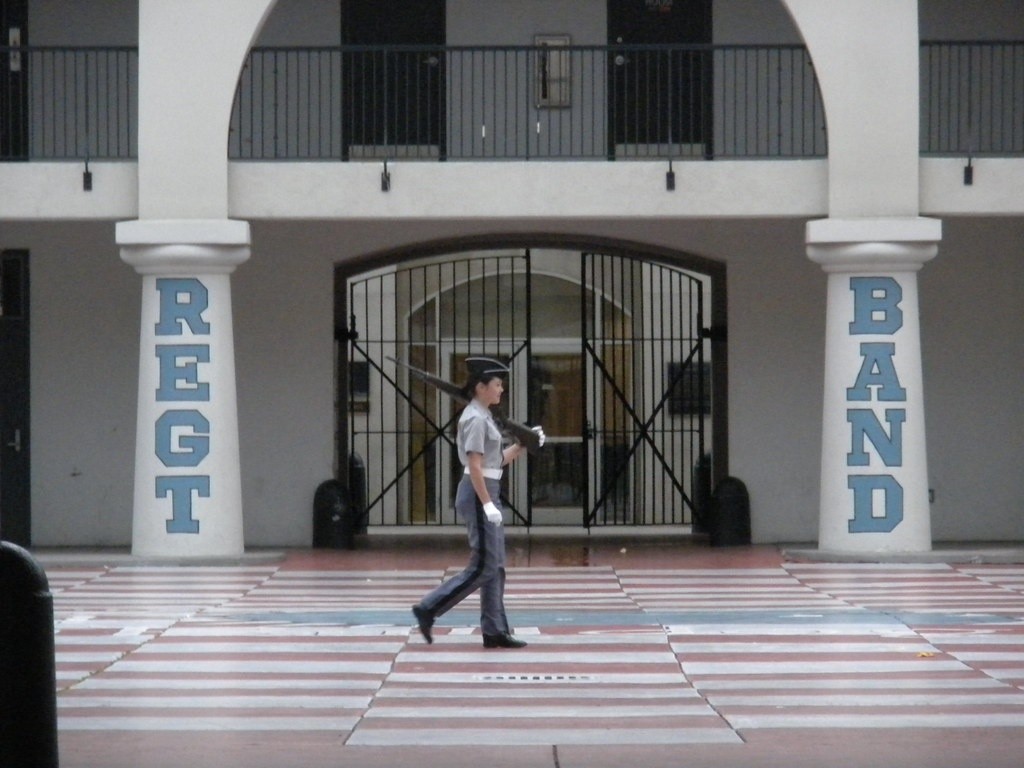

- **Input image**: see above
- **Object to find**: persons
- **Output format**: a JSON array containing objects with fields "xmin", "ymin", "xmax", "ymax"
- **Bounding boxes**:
[{"xmin": 409, "ymin": 357, "xmax": 531, "ymax": 650}]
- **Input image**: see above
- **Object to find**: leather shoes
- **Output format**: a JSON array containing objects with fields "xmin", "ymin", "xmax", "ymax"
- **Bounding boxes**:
[
  {"xmin": 483, "ymin": 631, "xmax": 528, "ymax": 648},
  {"xmin": 412, "ymin": 603, "xmax": 435, "ymax": 644}
]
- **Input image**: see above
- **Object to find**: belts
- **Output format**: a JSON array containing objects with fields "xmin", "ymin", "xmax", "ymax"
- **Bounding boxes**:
[{"xmin": 464, "ymin": 466, "xmax": 503, "ymax": 480}]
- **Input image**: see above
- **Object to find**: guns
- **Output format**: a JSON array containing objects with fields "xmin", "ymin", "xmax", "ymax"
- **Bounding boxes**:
[{"xmin": 384, "ymin": 355, "xmax": 540, "ymax": 451}]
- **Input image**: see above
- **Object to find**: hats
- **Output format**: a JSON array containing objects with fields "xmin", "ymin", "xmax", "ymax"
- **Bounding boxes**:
[{"xmin": 465, "ymin": 354, "xmax": 510, "ymax": 379}]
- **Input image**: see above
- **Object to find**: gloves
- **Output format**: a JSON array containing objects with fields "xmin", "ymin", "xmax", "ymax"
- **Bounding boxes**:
[
  {"xmin": 483, "ymin": 501, "xmax": 502, "ymax": 527},
  {"xmin": 531, "ymin": 426, "xmax": 545, "ymax": 448}
]
